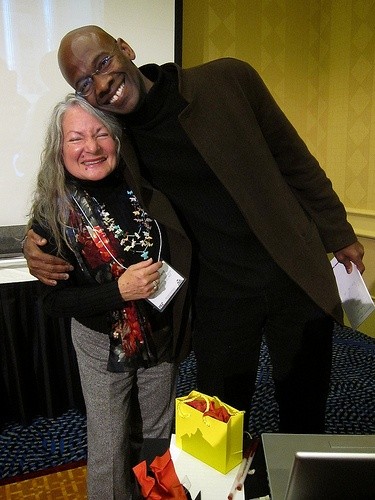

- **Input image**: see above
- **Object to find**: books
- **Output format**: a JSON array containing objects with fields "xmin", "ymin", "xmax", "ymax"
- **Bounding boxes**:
[{"xmin": 329, "ymin": 255, "xmax": 375, "ymax": 330}]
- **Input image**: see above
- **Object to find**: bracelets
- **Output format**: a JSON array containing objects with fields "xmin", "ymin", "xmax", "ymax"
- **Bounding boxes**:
[{"xmin": 19, "ymin": 234, "xmax": 27, "ymax": 254}]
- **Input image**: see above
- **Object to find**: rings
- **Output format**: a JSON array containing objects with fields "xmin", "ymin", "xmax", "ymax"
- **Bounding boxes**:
[{"xmin": 152, "ymin": 280, "xmax": 160, "ymax": 289}]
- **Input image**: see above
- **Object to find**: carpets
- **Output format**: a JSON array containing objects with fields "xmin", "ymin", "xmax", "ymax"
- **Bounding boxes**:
[{"xmin": 0, "ymin": 459, "xmax": 88, "ymax": 500}]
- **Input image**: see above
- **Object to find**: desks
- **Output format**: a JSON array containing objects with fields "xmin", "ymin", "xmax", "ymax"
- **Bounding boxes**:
[{"xmin": 0, "ymin": 256, "xmax": 85, "ymax": 427}]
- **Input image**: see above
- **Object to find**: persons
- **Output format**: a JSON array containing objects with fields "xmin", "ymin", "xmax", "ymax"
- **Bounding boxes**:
[
  {"xmin": 28, "ymin": 93, "xmax": 195, "ymax": 500},
  {"xmin": 18, "ymin": 24, "xmax": 366, "ymax": 480}
]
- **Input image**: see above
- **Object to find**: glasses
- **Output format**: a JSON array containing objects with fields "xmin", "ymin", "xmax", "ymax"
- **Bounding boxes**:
[{"xmin": 75, "ymin": 39, "xmax": 116, "ymax": 97}]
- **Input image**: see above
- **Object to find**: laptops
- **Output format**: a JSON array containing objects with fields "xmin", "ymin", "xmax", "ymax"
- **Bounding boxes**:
[{"xmin": 261, "ymin": 433, "xmax": 375, "ymax": 500}]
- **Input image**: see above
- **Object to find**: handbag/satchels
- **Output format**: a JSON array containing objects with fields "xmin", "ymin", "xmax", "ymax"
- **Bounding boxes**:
[{"xmin": 175, "ymin": 390, "xmax": 246, "ymax": 475}]
